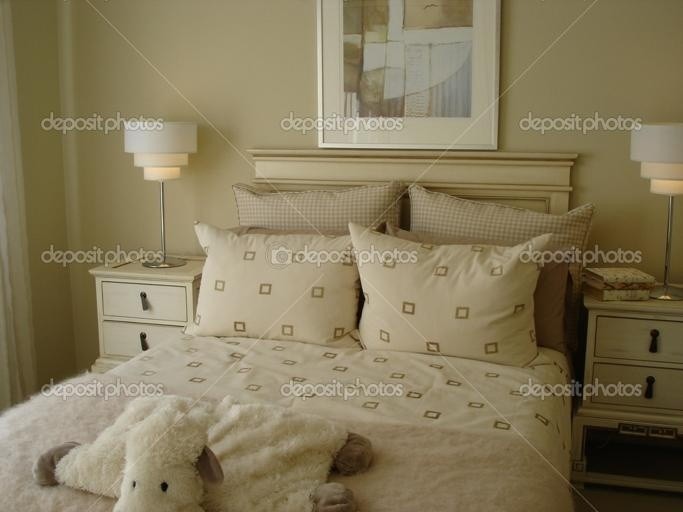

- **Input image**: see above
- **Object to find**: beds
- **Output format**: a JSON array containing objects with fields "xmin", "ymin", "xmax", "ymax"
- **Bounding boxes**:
[{"xmin": 0, "ymin": 149, "xmax": 595, "ymax": 512}]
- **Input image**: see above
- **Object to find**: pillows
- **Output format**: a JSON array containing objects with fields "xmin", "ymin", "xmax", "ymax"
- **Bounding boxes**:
[{"xmin": 182, "ymin": 178, "xmax": 596, "ymax": 370}]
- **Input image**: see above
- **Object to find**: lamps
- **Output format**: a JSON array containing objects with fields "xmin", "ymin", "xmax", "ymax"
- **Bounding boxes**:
[
  {"xmin": 123, "ymin": 117, "xmax": 198, "ymax": 269},
  {"xmin": 626, "ymin": 119, "xmax": 683, "ymax": 302}
]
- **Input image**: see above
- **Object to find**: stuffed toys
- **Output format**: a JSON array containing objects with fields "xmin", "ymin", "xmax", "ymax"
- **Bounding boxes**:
[{"xmin": 34, "ymin": 390, "xmax": 372, "ymax": 512}]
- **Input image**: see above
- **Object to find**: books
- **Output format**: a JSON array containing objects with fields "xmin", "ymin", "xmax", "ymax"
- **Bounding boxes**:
[{"xmin": 582, "ymin": 266, "xmax": 656, "ymax": 301}]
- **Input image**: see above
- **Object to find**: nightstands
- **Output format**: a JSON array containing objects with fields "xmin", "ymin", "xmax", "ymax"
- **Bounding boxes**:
[
  {"xmin": 569, "ymin": 281, "xmax": 682, "ymax": 495},
  {"xmin": 87, "ymin": 253, "xmax": 207, "ymax": 373}
]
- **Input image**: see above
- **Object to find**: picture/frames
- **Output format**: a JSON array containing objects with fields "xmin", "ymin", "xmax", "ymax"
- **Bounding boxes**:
[{"xmin": 314, "ymin": 1, "xmax": 503, "ymax": 152}]
